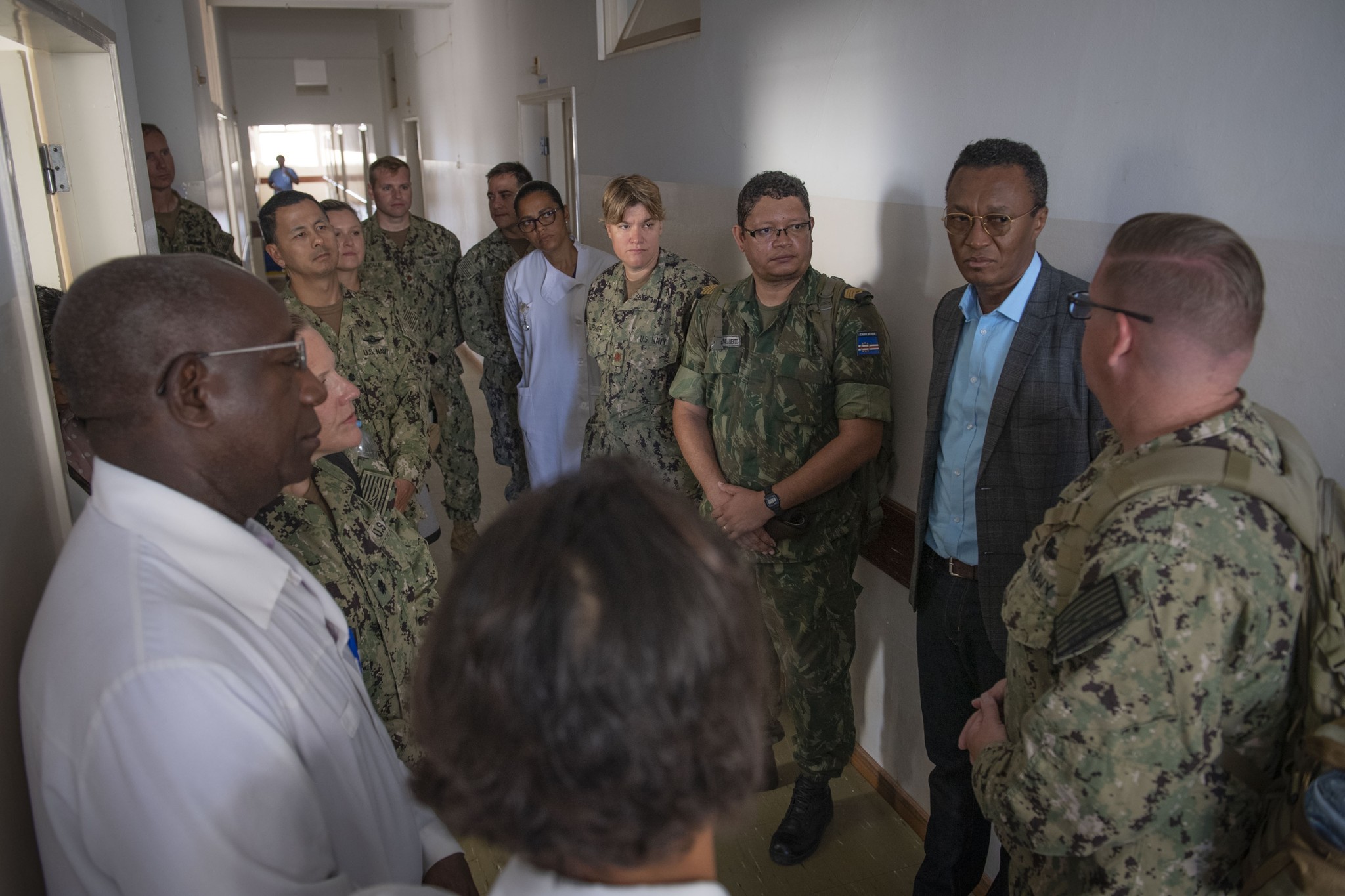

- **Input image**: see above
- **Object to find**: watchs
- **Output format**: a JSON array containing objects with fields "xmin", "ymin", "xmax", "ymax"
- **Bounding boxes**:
[{"xmin": 764, "ymin": 485, "xmax": 783, "ymax": 517}]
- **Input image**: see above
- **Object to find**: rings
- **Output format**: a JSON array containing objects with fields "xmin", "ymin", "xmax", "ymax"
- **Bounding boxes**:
[{"xmin": 724, "ymin": 525, "xmax": 731, "ymax": 533}]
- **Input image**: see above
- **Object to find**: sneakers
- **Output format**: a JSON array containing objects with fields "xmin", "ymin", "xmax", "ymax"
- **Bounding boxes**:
[
  {"xmin": 744, "ymin": 744, "xmax": 778, "ymax": 792},
  {"xmin": 770, "ymin": 776, "xmax": 835, "ymax": 867}
]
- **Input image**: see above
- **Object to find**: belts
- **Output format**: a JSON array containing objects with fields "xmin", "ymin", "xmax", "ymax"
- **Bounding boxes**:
[{"xmin": 921, "ymin": 544, "xmax": 978, "ymax": 581}]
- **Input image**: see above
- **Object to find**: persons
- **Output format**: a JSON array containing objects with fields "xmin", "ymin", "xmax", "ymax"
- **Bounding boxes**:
[
  {"xmin": 402, "ymin": 453, "xmax": 798, "ymax": 895},
  {"xmin": 667, "ymin": 170, "xmax": 893, "ymax": 865},
  {"xmin": 141, "ymin": 122, "xmax": 243, "ymax": 267},
  {"xmin": 905, "ymin": 137, "xmax": 1112, "ymax": 894},
  {"xmin": 256, "ymin": 189, "xmax": 432, "ymax": 536},
  {"xmin": 266, "ymin": 155, "xmax": 299, "ymax": 196},
  {"xmin": 231, "ymin": 308, "xmax": 442, "ymax": 760},
  {"xmin": 502, "ymin": 181, "xmax": 621, "ymax": 495},
  {"xmin": 451, "ymin": 162, "xmax": 537, "ymax": 499},
  {"xmin": 17, "ymin": 251, "xmax": 476, "ymax": 895},
  {"xmin": 320, "ymin": 199, "xmax": 367, "ymax": 293},
  {"xmin": 358, "ymin": 155, "xmax": 487, "ymax": 555},
  {"xmin": 957, "ymin": 213, "xmax": 1320, "ymax": 894},
  {"xmin": 577, "ymin": 176, "xmax": 727, "ymax": 505}
]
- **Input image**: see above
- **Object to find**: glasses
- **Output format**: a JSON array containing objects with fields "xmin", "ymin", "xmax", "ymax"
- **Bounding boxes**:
[
  {"xmin": 517, "ymin": 207, "xmax": 561, "ymax": 234},
  {"xmin": 941, "ymin": 205, "xmax": 1037, "ymax": 237},
  {"xmin": 1066, "ymin": 290, "xmax": 1153, "ymax": 324},
  {"xmin": 740, "ymin": 220, "xmax": 812, "ymax": 243},
  {"xmin": 156, "ymin": 337, "xmax": 306, "ymax": 396}
]
